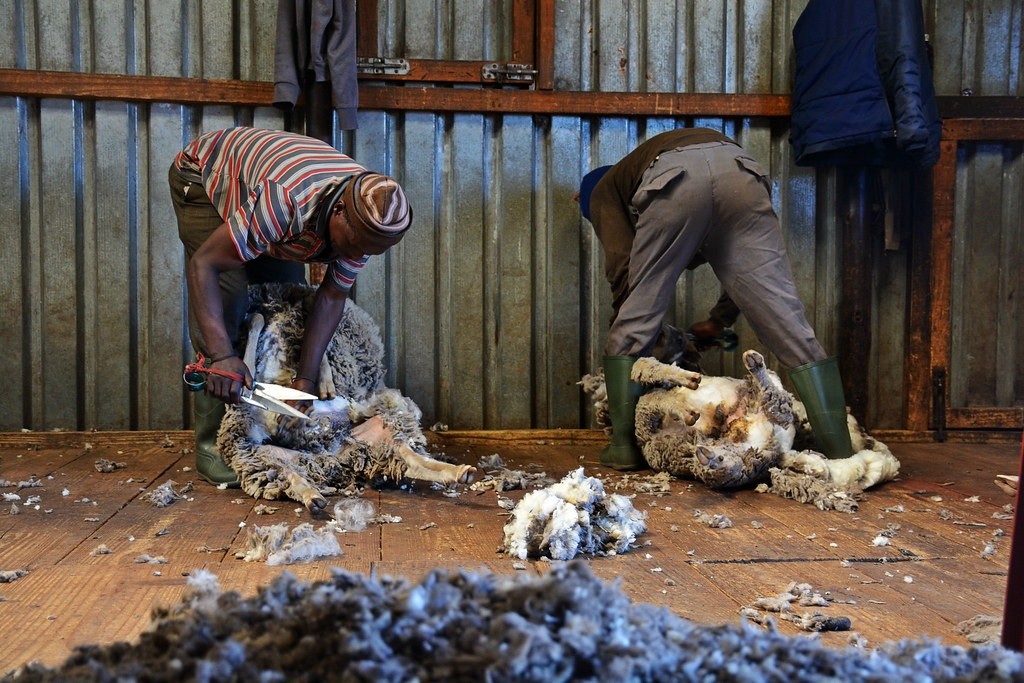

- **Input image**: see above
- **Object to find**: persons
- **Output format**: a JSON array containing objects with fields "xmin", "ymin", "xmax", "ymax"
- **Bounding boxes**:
[
  {"xmin": 579, "ymin": 126, "xmax": 856, "ymax": 470},
  {"xmin": 167, "ymin": 126, "xmax": 413, "ymax": 489}
]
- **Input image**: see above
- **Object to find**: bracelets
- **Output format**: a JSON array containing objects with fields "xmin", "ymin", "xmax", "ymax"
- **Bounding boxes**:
[
  {"xmin": 292, "ymin": 377, "xmax": 317, "ymax": 385},
  {"xmin": 211, "ymin": 351, "xmax": 239, "ymax": 365}
]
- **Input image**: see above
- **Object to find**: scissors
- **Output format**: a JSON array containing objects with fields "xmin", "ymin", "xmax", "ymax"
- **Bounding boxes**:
[
  {"xmin": 663, "ymin": 321, "xmax": 739, "ymax": 352},
  {"xmin": 180, "ymin": 360, "xmax": 320, "ymax": 420}
]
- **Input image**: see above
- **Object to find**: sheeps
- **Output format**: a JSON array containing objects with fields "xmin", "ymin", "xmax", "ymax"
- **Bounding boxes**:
[
  {"xmin": 216, "ymin": 281, "xmax": 477, "ymax": 515},
  {"xmin": 581, "ymin": 348, "xmax": 900, "ymax": 512}
]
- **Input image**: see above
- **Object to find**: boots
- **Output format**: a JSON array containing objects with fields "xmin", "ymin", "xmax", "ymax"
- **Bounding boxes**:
[
  {"xmin": 788, "ymin": 357, "xmax": 854, "ymax": 459},
  {"xmin": 195, "ymin": 357, "xmax": 240, "ymax": 490},
  {"xmin": 599, "ymin": 356, "xmax": 644, "ymax": 470}
]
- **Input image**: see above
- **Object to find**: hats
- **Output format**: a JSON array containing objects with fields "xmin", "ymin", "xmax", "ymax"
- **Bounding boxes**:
[
  {"xmin": 580, "ymin": 165, "xmax": 612, "ymax": 222},
  {"xmin": 344, "ymin": 171, "xmax": 413, "ymax": 255}
]
